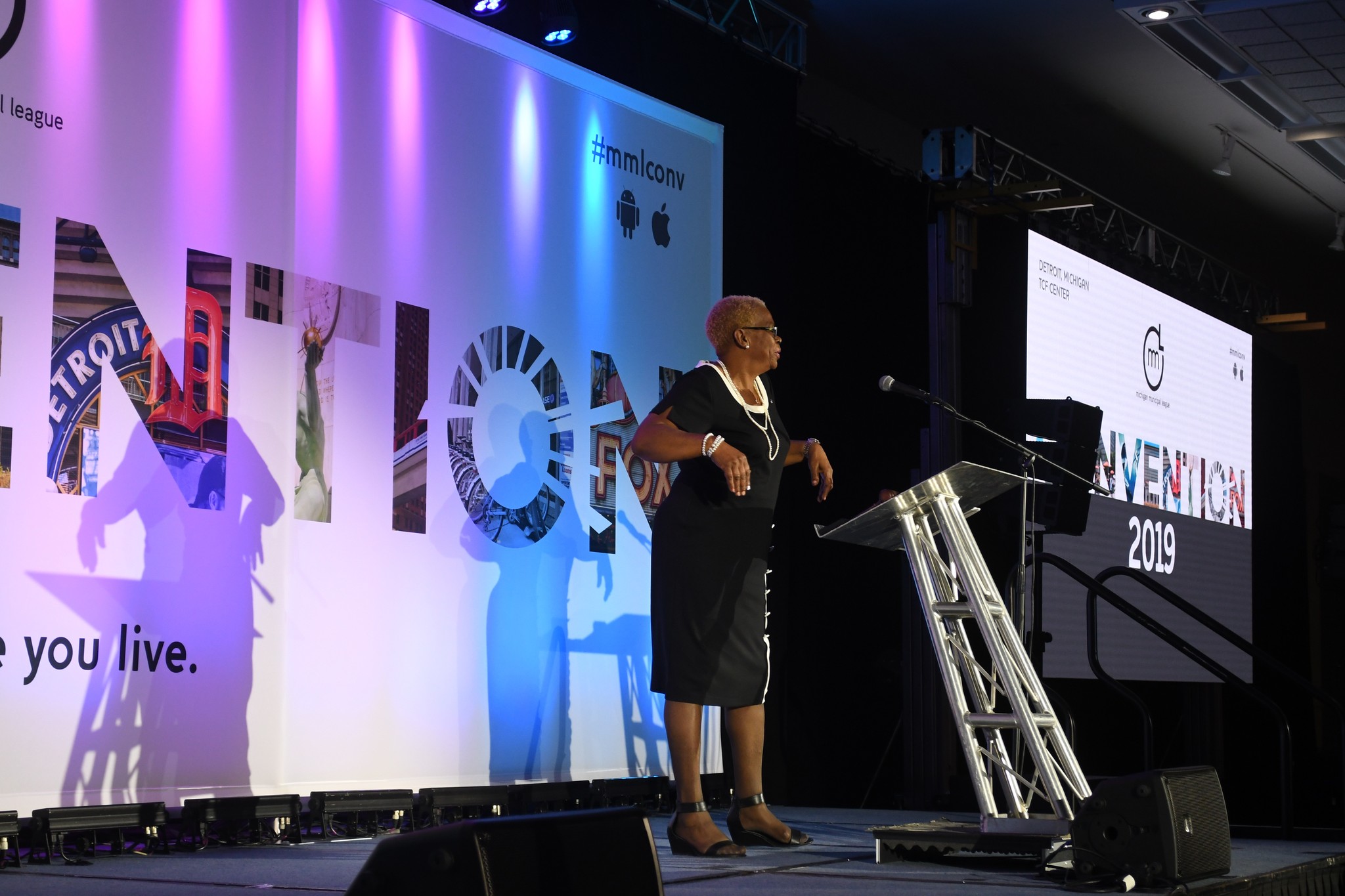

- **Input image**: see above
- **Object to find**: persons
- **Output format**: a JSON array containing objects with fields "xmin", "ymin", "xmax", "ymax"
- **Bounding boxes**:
[
  {"xmin": 633, "ymin": 297, "xmax": 834, "ymax": 858},
  {"xmin": 294, "ymin": 340, "xmax": 328, "ymax": 523}
]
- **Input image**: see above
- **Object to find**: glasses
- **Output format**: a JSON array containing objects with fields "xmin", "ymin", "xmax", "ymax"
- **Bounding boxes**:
[{"xmin": 741, "ymin": 326, "xmax": 778, "ymax": 338}]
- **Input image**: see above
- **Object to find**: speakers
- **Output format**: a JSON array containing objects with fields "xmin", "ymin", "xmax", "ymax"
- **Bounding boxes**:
[
  {"xmin": 1068, "ymin": 764, "xmax": 1233, "ymax": 885},
  {"xmin": 341, "ymin": 806, "xmax": 664, "ymax": 896},
  {"xmin": 1013, "ymin": 398, "xmax": 1104, "ymax": 535}
]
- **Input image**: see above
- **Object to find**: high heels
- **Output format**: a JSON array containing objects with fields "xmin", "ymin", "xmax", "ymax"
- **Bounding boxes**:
[
  {"xmin": 725, "ymin": 794, "xmax": 814, "ymax": 847},
  {"xmin": 666, "ymin": 802, "xmax": 747, "ymax": 860}
]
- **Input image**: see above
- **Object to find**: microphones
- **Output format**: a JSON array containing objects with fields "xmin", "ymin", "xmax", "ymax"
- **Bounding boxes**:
[{"xmin": 879, "ymin": 375, "xmax": 941, "ymax": 403}]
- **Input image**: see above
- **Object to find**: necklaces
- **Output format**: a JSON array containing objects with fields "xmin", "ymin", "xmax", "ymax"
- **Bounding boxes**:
[{"xmin": 716, "ymin": 358, "xmax": 780, "ymax": 460}]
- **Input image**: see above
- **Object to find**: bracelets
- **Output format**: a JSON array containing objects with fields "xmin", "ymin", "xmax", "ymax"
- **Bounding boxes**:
[
  {"xmin": 702, "ymin": 432, "xmax": 725, "ymax": 457},
  {"xmin": 803, "ymin": 438, "xmax": 821, "ymax": 459}
]
